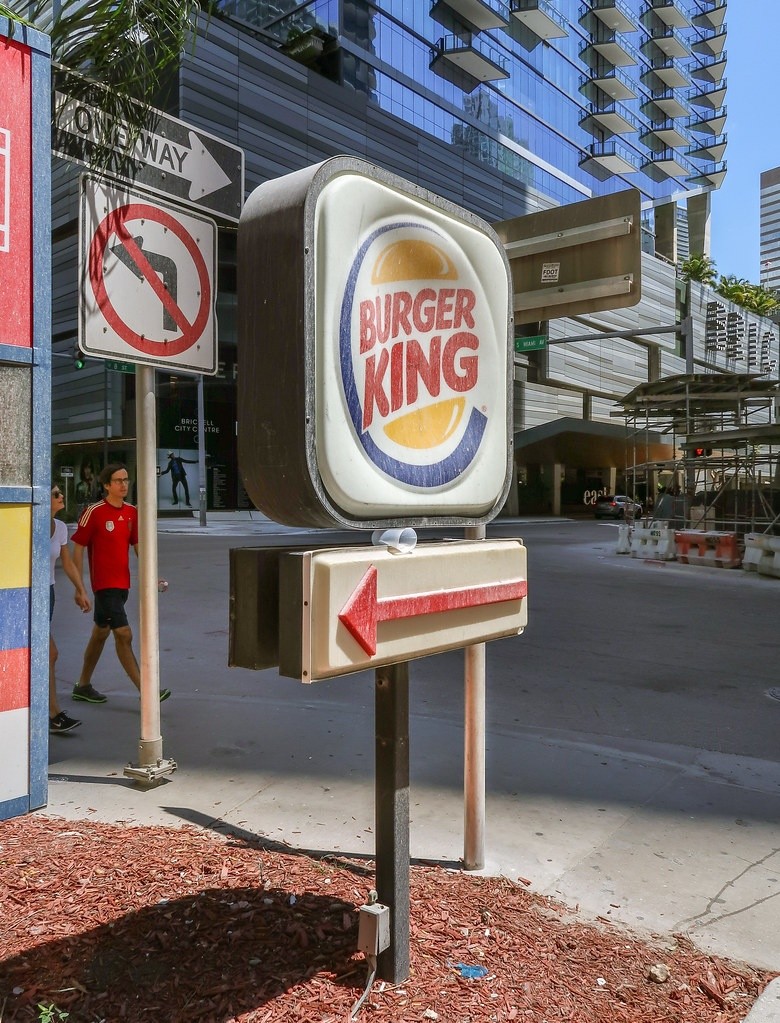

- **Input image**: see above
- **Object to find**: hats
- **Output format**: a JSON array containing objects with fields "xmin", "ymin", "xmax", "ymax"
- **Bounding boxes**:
[{"xmin": 167, "ymin": 450, "xmax": 174, "ymax": 458}]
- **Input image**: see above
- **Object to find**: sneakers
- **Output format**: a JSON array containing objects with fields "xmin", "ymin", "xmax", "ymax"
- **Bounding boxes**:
[
  {"xmin": 48, "ymin": 709, "xmax": 82, "ymax": 733},
  {"xmin": 160, "ymin": 688, "xmax": 172, "ymax": 702},
  {"xmin": 71, "ymin": 681, "xmax": 109, "ymax": 702}
]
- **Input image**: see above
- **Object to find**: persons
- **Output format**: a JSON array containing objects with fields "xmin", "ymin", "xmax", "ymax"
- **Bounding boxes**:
[
  {"xmin": 49, "ymin": 481, "xmax": 91, "ymax": 732},
  {"xmin": 75, "ymin": 458, "xmax": 96, "ymax": 503},
  {"xmin": 161, "ymin": 450, "xmax": 199, "ymax": 508},
  {"xmin": 70, "ymin": 465, "xmax": 171, "ymax": 704}
]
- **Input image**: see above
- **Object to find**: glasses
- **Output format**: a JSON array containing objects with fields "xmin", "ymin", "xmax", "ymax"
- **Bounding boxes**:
[
  {"xmin": 110, "ymin": 477, "xmax": 131, "ymax": 485},
  {"xmin": 51, "ymin": 489, "xmax": 62, "ymax": 499}
]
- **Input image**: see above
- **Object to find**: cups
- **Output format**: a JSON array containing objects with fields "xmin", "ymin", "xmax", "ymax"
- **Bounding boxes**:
[{"xmin": 371, "ymin": 528, "xmax": 417, "ymax": 554}]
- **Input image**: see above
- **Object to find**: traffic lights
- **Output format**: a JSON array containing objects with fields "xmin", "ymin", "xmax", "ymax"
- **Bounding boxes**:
[{"xmin": 694, "ymin": 448, "xmax": 704, "ymax": 456}]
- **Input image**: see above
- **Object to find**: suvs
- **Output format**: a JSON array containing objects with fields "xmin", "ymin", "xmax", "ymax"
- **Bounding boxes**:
[{"xmin": 592, "ymin": 495, "xmax": 643, "ymax": 520}]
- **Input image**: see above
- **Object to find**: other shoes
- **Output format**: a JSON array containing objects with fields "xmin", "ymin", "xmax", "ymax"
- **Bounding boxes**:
[
  {"xmin": 187, "ymin": 503, "xmax": 192, "ymax": 508},
  {"xmin": 172, "ymin": 501, "xmax": 179, "ymax": 505}
]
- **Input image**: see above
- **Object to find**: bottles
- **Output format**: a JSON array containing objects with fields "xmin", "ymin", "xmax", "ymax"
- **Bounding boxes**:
[{"xmin": 158, "ymin": 581, "xmax": 168, "ymax": 592}]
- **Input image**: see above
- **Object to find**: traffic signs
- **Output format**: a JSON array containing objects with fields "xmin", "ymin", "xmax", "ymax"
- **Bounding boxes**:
[{"xmin": 47, "ymin": 60, "xmax": 246, "ymax": 221}]
